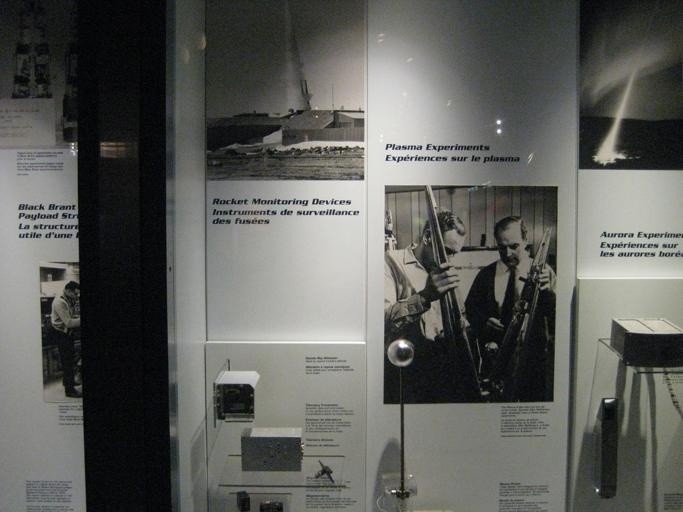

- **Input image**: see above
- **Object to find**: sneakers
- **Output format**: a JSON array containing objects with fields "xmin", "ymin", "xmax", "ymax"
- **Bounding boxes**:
[{"xmin": 63, "ymin": 380, "xmax": 83, "ymax": 397}]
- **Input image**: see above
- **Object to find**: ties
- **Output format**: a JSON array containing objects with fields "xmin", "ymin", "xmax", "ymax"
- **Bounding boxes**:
[{"xmin": 501, "ymin": 265, "xmax": 516, "ymax": 333}]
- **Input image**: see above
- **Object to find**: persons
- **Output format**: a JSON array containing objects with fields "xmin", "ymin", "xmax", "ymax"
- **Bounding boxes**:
[
  {"xmin": 49, "ymin": 280, "xmax": 84, "ymax": 399},
  {"xmin": 462, "ymin": 215, "xmax": 556, "ymax": 402},
  {"xmin": 384, "ymin": 212, "xmax": 480, "ymax": 402}
]
